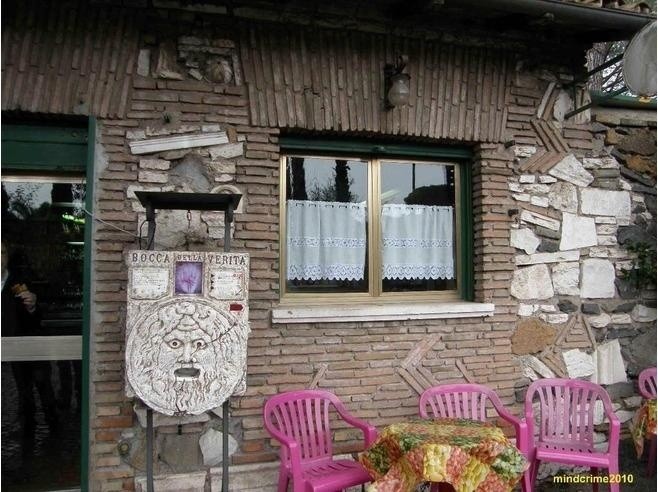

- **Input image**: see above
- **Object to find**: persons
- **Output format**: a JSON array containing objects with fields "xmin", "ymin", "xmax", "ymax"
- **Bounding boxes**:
[
  {"xmin": 1, "ymin": 240, "xmax": 56, "ymax": 337},
  {"xmin": 11, "ymin": 361, "xmax": 57, "ymax": 465},
  {"xmin": 58, "ymin": 361, "xmax": 83, "ymax": 417}
]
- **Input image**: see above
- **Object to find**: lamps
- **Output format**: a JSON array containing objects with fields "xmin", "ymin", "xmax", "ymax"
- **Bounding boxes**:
[{"xmin": 385, "ymin": 71, "xmax": 410, "ymax": 104}]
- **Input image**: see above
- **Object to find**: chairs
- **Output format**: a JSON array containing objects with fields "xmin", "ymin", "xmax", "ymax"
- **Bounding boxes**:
[
  {"xmin": 639, "ymin": 367, "xmax": 657, "ymax": 476},
  {"xmin": 264, "ymin": 389, "xmax": 379, "ymax": 492},
  {"xmin": 526, "ymin": 378, "xmax": 619, "ymax": 492},
  {"xmin": 423, "ymin": 384, "xmax": 532, "ymax": 491}
]
